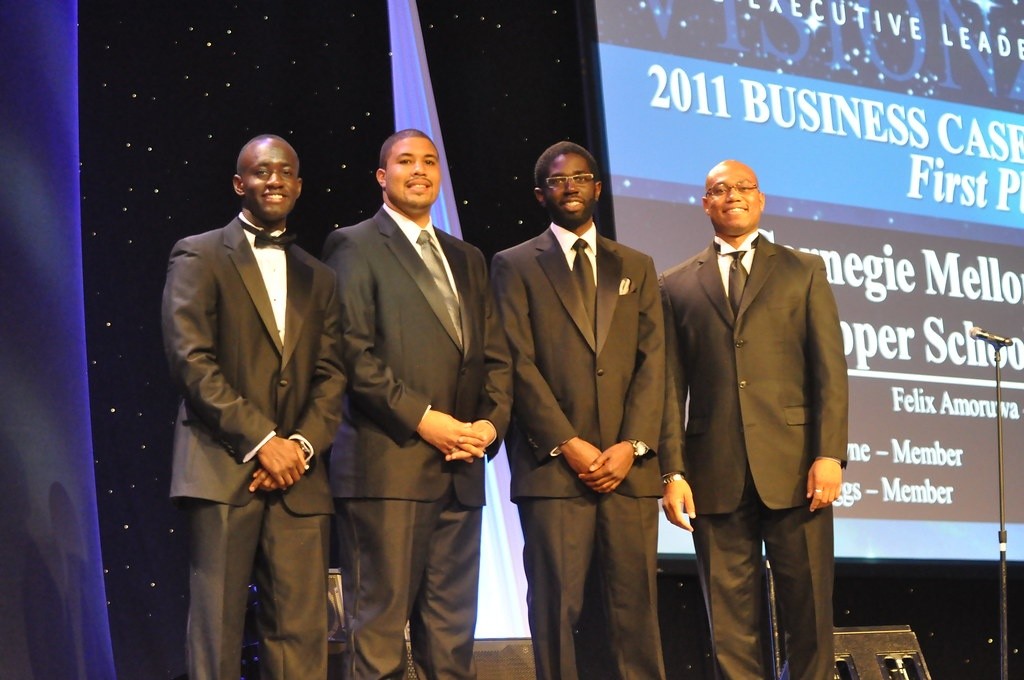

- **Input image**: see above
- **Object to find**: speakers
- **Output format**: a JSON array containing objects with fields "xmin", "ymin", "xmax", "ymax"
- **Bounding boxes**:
[{"xmin": 834, "ymin": 625, "xmax": 933, "ymax": 680}]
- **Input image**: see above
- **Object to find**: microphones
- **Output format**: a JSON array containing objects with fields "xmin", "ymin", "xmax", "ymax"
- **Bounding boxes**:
[{"xmin": 969, "ymin": 327, "xmax": 1013, "ymax": 347}]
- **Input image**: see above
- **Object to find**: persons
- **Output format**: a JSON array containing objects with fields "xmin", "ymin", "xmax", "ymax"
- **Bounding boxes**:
[
  {"xmin": 162, "ymin": 134, "xmax": 347, "ymax": 680},
  {"xmin": 492, "ymin": 140, "xmax": 665, "ymax": 680},
  {"xmin": 658, "ymin": 158, "xmax": 849, "ymax": 680},
  {"xmin": 321, "ymin": 129, "xmax": 515, "ymax": 680}
]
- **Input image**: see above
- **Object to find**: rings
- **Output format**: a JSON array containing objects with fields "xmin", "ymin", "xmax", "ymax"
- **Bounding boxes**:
[
  {"xmin": 815, "ymin": 490, "xmax": 823, "ymax": 492},
  {"xmin": 453, "ymin": 447, "xmax": 457, "ymax": 453}
]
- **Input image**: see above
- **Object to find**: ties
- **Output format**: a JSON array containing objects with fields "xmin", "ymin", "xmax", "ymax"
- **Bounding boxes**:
[
  {"xmin": 416, "ymin": 230, "xmax": 464, "ymax": 350},
  {"xmin": 712, "ymin": 236, "xmax": 759, "ymax": 322},
  {"xmin": 571, "ymin": 238, "xmax": 597, "ymax": 341}
]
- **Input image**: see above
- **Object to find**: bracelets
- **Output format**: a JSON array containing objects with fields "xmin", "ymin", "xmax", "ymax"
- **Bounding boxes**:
[
  {"xmin": 664, "ymin": 473, "xmax": 684, "ymax": 484},
  {"xmin": 300, "ymin": 441, "xmax": 311, "ymax": 453}
]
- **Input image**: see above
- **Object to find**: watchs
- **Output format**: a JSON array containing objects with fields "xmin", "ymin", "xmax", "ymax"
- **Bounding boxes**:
[{"xmin": 631, "ymin": 439, "xmax": 648, "ymax": 457}]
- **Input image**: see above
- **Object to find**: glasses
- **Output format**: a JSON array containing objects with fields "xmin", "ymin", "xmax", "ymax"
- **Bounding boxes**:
[
  {"xmin": 705, "ymin": 180, "xmax": 758, "ymax": 200},
  {"xmin": 540, "ymin": 173, "xmax": 594, "ymax": 189}
]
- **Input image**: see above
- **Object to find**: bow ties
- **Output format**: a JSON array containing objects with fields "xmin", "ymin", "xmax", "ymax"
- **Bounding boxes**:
[{"xmin": 237, "ymin": 217, "xmax": 298, "ymax": 252}]
